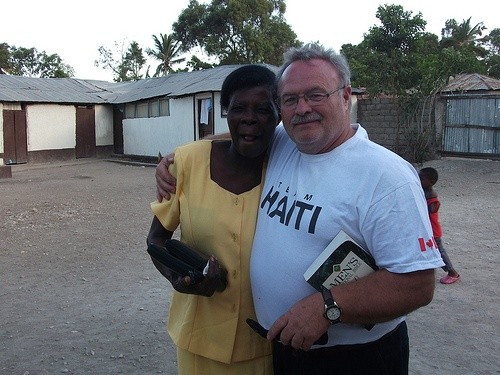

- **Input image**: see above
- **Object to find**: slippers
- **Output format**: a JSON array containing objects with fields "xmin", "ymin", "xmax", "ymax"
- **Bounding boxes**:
[{"xmin": 441, "ymin": 273, "xmax": 462, "ymax": 284}]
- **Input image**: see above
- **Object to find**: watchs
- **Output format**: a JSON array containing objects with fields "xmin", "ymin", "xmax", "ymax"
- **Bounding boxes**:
[{"xmin": 320, "ymin": 289, "xmax": 343, "ymax": 326}]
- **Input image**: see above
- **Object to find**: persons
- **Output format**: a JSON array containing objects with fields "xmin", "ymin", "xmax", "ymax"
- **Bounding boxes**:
[
  {"xmin": 155, "ymin": 42, "xmax": 447, "ymax": 375},
  {"xmin": 416, "ymin": 167, "xmax": 462, "ymax": 284},
  {"xmin": 144, "ymin": 64, "xmax": 283, "ymax": 375}
]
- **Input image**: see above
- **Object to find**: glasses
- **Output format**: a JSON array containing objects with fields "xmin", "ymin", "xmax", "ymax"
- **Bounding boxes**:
[{"xmin": 276, "ymin": 87, "xmax": 345, "ymax": 110}]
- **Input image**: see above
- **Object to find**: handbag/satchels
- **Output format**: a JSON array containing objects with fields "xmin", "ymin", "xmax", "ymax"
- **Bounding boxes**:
[{"xmin": 147, "ymin": 239, "xmax": 228, "ymax": 292}]
